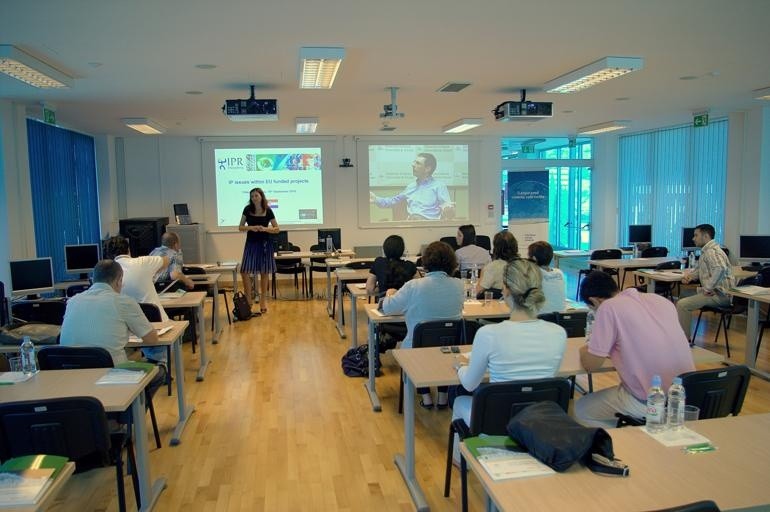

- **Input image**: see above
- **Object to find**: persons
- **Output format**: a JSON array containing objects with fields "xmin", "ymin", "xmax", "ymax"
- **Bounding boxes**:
[
  {"xmin": 102, "ymin": 235, "xmax": 171, "ymax": 323},
  {"xmin": 570, "ymin": 270, "xmax": 695, "ymax": 428},
  {"xmin": 58, "ymin": 258, "xmax": 166, "ymax": 413},
  {"xmin": 456, "ymin": 225, "xmax": 492, "ymax": 269},
  {"xmin": 238, "ymin": 187, "xmax": 279, "ymax": 314},
  {"xmin": 366, "ymin": 235, "xmax": 422, "ymax": 297},
  {"xmin": 453, "ymin": 262, "xmax": 567, "ymax": 470},
  {"xmin": 503, "ymin": 241, "xmax": 565, "ymax": 313},
  {"xmin": 476, "ymin": 230, "xmax": 521, "ymax": 323},
  {"xmin": 675, "ymin": 223, "xmax": 736, "ymax": 346},
  {"xmin": 370, "ymin": 152, "xmax": 456, "ymax": 221},
  {"xmin": 147, "ymin": 231, "xmax": 195, "ymax": 290},
  {"xmin": 383, "ymin": 241, "xmax": 466, "ymax": 410}
]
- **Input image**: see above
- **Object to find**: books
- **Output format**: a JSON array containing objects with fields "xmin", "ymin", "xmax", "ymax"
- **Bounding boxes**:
[
  {"xmin": 96, "ymin": 361, "xmax": 156, "ymax": 385},
  {"xmin": 160, "ymin": 291, "xmax": 186, "ymax": 299},
  {"xmin": 463, "ymin": 436, "xmax": 557, "ymax": 482},
  {"xmin": 0, "ymin": 454, "xmax": 78, "ymax": 506}
]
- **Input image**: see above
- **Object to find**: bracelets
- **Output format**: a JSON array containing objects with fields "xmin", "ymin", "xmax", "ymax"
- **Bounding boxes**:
[
  {"xmin": 456, "ymin": 362, "xmax": 465, "ymax": 372},
  {"xmin": 388, "ymin": 294, "xmax": 392, "ymax": 296}
]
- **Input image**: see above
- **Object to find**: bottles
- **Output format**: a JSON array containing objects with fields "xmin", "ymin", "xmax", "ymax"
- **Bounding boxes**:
[
  {"xmin": 666, "ymin": 378, "xmax": 686, "ymax": 431},
  {"xmin": 177, "ymin": 249, "xmax": 184, "ymax": 265},
  {"xmin": 632, "ymin": 242, "xmax": 638, "ymax": 258},
  {"xmin": 645, "ymin": 374, "xmax": 667, "ymax": 434},
  {"xmin": 461, "ymin": 262, "xmax": 479, "ymax": 303},
  {"xmin": 583, "ymin": 309, "xmax": 596, "ymax": 346},
  {"xmin": 20, "ymin": 336, "xmax": 36, "ymax": 374},
  {"xmin": 688, "ymin": 252, "xmax": 695, "ymax": 270}
]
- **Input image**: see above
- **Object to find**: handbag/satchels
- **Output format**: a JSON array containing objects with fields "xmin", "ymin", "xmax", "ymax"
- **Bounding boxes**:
[
  {"xmin": 233, "ymin": 291, "xmax": 251, "ymax": 321},
  {"xmin": 507, "ymin": 400, "xmax": 614, "ymax": 471},
  {"xmin": 342, "ymin": 344, "xmax": 368, "ymax": 377}
]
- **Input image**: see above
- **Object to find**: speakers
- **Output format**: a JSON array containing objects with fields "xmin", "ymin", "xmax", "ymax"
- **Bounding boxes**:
[{"xmin": 119, "ymin": 217, "xmax": 170, "ymax": 258}]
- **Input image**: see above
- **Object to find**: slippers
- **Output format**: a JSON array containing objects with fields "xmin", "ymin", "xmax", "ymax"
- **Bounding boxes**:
[
  {"xmin": 420, "ymin": 400, "xmax": 433, "ymax": 408},
  {"xmin": 437, "ymin": 400, "xmax": 448, "ymax": 408}
]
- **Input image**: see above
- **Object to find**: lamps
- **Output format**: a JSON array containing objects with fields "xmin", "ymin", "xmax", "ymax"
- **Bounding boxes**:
[
  {"xmin": 121, "ymin": 118, "xmax": 166, "ymax": 135},
  {"xmin": 441, "ymin": 118, "xmax": 483, "ymax": 136},
  {"xmin": 751, "ymin": 88, "xmax": 770, "ymax": 101},
  {"xmin": 520, "ymin": 139, "xmax": 546, "ymax": 146},
  {"xmin": 579, "ymin": 121, "xmax": 631, "ymax": 136},
  {"xmin": 295, "ymin": 117, "xmax": 317, "ymax": 133},
  {"xmin": 544, "ymin": 57, "xmax": 643, "ymax": 96},
  {"xmin": 0, "ymin": 45, "xmax": 75, "ymax": 91},
  {"xmin": 298, "ymin": 47, "xmax": 345, "ymax": 91}
]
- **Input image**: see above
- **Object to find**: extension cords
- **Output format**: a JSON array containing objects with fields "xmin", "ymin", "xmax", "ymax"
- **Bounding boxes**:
[{"xmin": 315, "ymin": 295, "xmax": 332, "ymax": 300}]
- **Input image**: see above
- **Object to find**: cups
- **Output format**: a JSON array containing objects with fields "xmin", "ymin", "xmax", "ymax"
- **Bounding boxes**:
[
  {"xmin": 679, "ymin": 405, "xmax": 701, "ymax": 440},
  {"xmin": 662, "ymin": 407, "xmax": 681, "ymax": 442},
  {"xmin": 484, "ymin": 291, "xmax": 493, "ymax": 304},
  {"xmin": 8, "ymin": 357, "xmax": 24, "ymax": 380}
]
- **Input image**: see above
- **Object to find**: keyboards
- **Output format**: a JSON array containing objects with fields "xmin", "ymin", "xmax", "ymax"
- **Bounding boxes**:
[
  {"xmin": 279, "ymin": 251, "xmax": 293, "ymax": 254},
  {"xmin": 312, "ymin": 250, "xmax": 327, "ymax": 252}
]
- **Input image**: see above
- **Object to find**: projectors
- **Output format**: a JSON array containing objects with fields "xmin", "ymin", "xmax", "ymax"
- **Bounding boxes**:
[
  {"xmin": 222, "ymin": 99, "xmax": 279, "ymax": 122},
  {"xmin": 495, "ymin": 102, "xmax": 554, "ymax": 122}
]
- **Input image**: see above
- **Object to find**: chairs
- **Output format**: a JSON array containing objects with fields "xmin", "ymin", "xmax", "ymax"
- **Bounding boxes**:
[
  {"xmin": 443, "ymin": 375, "xmax": 578, "ymax": 511},
  {"xmin": 12, "ymin": 301, "xmax": 173, "ymax": 396},
  {"xmin": 2, "ymin": 346, "xmax": 163, "ymax": 449},
  {"xmin": 309, "ymin": 245, "xmax": 342, "ymax": 299},
  {"xmin": 0, "ymin": 395, "xmax": 142, "ymax": 512},
  {"xmin": 614, "ymin": 361, "xmax": 753, "ymax": 431},
  {"xmin": 67, "ymin": 267, "xmax": 232, "ymax": 353},
  {"xmin": 377, "ymin": 268, "xmax": 595, "ymax": 414},
  {"xmin": 576, "ymin": 245, "xmax": 769, "ymax": 364},
  {"xmin": 271, "ymin": 246, "xmax": 308, "ymax": 299},
  {"xmin": 330, "ymin": 255, "xmax": 431, "ymax": 320}
]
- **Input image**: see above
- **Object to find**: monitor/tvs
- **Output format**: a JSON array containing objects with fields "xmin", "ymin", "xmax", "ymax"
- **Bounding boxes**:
[
  {"xmin": 318, "ymin": 228, "xmax": 341, "ymax": 252},
  {"xmin": 681, "ymin": 227, "xmax": 702, "ymax": 260},
  {"xmin": 64, "ymin": 244, "xmax": 99, "ymax": 281},
  {"xmin": 629, "ymin": 225, "xmax": 652, "ymax": 245},
  {"xmin": 271, "ymin": 231, "xmax": 289, "ymax": 256},
  {"xmin": 9, "ymin": 257, "xmax": 55, "ymax": 302},
  {"xmin": 737, "ymin": 234, "xmax": 770, "ymax": 270}
]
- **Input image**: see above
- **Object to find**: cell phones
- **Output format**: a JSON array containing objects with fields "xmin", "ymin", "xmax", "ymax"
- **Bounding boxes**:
[
  {"xmin": 441, "ymin": 347, "xmax": 451, "ymax": 353},
  {"xmin": 451, "ymin": 347, "xmax": 460, "ymax": 353}
]
